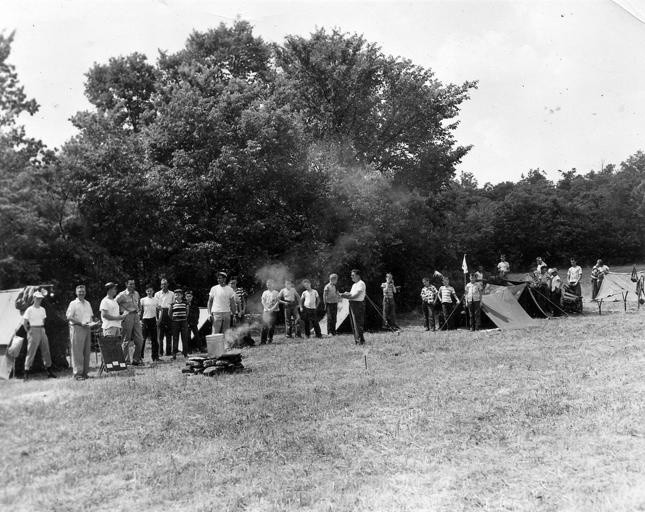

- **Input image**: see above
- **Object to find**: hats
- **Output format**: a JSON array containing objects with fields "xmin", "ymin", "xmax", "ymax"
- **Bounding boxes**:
[
  {"xmin": 105, "ymin": 282, "xmax": 118, "ymax": 289},
  {"xmin": 32, "ymin": 292, "xmax": 44, "ymax": 298}
]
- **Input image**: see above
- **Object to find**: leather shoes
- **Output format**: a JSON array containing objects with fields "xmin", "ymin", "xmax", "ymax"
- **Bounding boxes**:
[
  {"xmin": 24, "ymin": 371, "xmax": 29, "ymax": 380},
  {"xmin": 47, "ymin": 367, "xmax": 57, "ymax": 377}
]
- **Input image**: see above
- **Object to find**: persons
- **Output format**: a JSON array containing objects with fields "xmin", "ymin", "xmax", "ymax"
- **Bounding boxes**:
[
  {"xmin": 22, "ymin": 291, "xmax": 58, "ymax": 382},
  {"xmin": 64, "ymin": 284, "xmax": 97, "ymax": 382},
  {"xmin": 98, "ymin": 255, "xmax": 609, "ymax": 367}
]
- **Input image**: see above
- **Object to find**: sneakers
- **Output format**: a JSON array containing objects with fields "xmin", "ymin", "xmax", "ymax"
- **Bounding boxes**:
[
  {"xmin": 131, "ymin": 354, "xmax": 163, "ymax": 366},
  {"xmin": 165, "ymin": 351, "xmax": 206, "ymax": 360},
  {"xmin": 76, "ymin": 375, "xmax": 94, "ymax": 380}
]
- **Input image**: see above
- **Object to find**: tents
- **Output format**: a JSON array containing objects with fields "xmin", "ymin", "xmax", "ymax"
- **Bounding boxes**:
[
  {"xmin": 591, "ymin": 269, "xmax": 645, "ymax": 302},
  {"xmin": 0, "ymin": 285, "xmax": 69, "ymax": 380}
]
